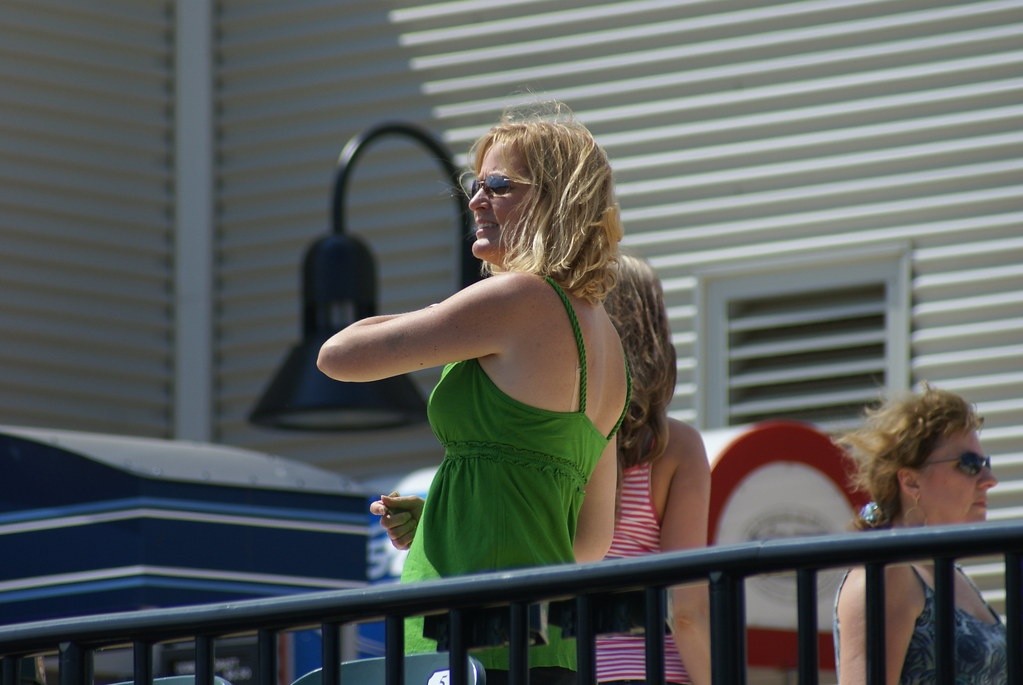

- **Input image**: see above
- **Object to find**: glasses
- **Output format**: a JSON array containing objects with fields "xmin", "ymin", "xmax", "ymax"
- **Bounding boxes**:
[
  {"xmin": 472, "ymin": 174, "xmax": 538, "ymax": 199},
  {"xmin": 923, "ymin": 452, "xmax": 991, "ymax": 476}
]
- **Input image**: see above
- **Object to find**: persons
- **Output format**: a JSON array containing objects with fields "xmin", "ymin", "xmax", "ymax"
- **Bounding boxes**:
[
  {"xmin": 315, "ymin": 120, "xmax": 632, "ymax": 685},
  {"xmin": 593, "ymin": 255, "xmax": 712, "ymax": 685},
  {"xmin": 832, "ymin": 389, "xmax": 1005, "ymax": 685}
]
